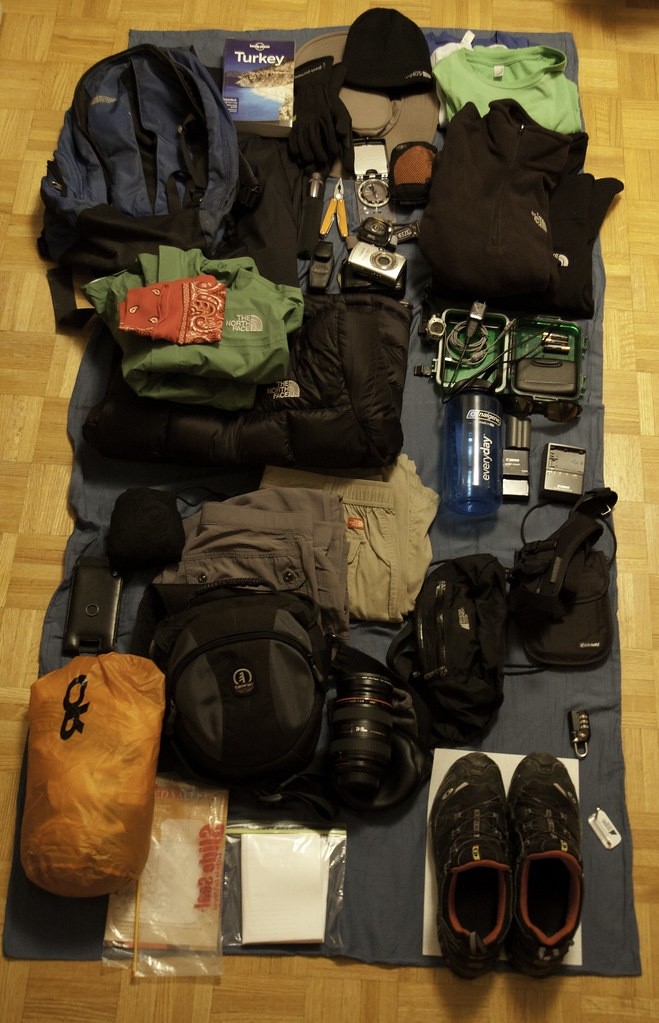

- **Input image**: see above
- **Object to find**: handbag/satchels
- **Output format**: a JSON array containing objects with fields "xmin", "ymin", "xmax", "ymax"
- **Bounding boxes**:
[
  {"xmin": 108, "ymin": 486, "xmax": 186, "ymax": 569},
  {"xmin": 20, "ymin": 650, "xmax": 163, "ymax": 898},
  {"xmin": 506, "ymin": 486, "xmax": 615, "ymax": 667},
  {"xmin": 388, "ymin": 551, "xmax": 511, "ymax": 741}
]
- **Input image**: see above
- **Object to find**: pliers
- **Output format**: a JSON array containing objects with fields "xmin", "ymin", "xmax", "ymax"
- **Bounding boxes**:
[{"xmin": 319, "ymin": 178, "xmax": 349, "ymax": 239}]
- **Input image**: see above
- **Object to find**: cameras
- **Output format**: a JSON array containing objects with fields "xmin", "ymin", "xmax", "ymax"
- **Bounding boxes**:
[{"xmin": 347, "ymin": 241, "xmax": 407, "ymax": 288}]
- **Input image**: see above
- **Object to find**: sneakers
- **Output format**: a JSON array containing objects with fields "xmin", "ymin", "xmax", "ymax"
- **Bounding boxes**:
[
  {"xmin": 430, "ymin": 753, "xmax": 515, "ymax": 978},
  {"xmin": 508, "ymin": 750, "xmax": 581, "ymax": 976}
]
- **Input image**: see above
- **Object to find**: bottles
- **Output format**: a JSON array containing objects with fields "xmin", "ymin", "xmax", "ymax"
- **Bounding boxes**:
[{"xmin": 441, "ymin": 378, "xmax": 503, "ymax": 516}]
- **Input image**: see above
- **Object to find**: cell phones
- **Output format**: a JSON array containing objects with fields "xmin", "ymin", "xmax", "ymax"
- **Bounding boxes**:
[{"xmin": 61, "ymin": 564, "xmax": 122, "ymax": 655}]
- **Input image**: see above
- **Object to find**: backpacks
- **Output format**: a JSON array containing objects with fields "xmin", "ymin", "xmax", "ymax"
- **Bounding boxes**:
[
  {"xmin": 35, "ymin": 46, "xmax": 240, "ymax": 272},
  {"xmin": 145, "ymin": 575, "xmax": 323, "ymax": 803}
]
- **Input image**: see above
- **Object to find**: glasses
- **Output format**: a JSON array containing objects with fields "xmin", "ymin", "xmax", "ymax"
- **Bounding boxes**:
[{"xmin": 502, "ymin": 392, "xmax": 585, "ymax": 422}]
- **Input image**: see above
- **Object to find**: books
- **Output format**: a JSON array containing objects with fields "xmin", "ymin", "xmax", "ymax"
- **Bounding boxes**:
[{"xmin": 220, "ymin": 38, "xmax": 295, "ymax": 138}]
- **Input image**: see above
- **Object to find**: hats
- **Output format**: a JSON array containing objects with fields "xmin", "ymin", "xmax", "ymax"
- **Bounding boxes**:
[{"xmin": 330, "ymin": 8, "xmax": 434, "ymax": 96}]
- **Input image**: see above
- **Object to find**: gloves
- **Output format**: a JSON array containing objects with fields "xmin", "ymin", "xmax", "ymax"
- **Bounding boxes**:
[{"xmin": 288, "ymin": 55, "xmax": 354, "ymax": 174}]
